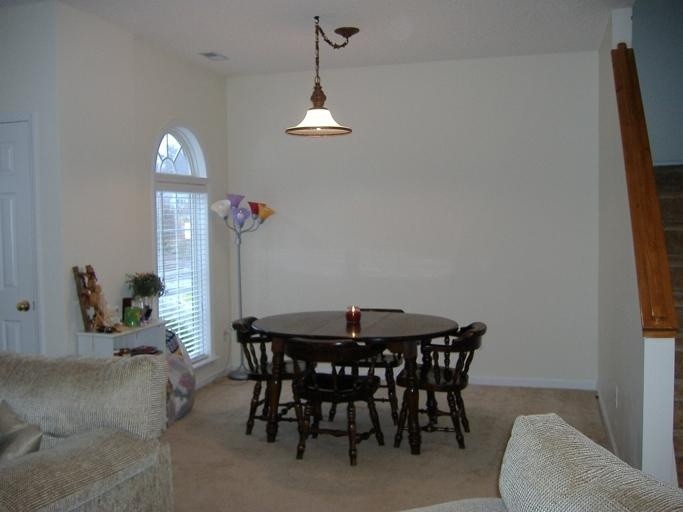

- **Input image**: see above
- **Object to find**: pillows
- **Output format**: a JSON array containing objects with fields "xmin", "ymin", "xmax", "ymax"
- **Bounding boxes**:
[{"xmin": 1, "ymin": 399, "xmax": 44, "ymax": 463}]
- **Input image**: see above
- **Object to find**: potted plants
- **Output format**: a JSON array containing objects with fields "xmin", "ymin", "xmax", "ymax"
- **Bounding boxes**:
[{"xmin": 124, "ymin": 270, "xmax": 165, "ymax": 326}]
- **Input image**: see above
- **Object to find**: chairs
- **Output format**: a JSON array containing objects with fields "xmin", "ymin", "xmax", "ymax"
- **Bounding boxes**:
[
  {"xmin": 405, "ymin": 413, "xmax": 683, "ymax": 511},
  {"xmin": 0, "ymin": 347, "xmax": 174, "ymax": 512}
]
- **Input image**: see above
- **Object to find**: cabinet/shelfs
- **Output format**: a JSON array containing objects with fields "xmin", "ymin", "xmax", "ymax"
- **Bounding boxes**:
[{"xmin": 75, "ymin": 319, "xmax": 171, "ymax": 356}]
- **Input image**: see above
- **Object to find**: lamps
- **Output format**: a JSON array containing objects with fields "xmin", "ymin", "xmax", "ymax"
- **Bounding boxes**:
[
  {"xmin": 210, "ymin": 193, "xmax": 276, "ymax": 380},
  {"xmin": 285, "ymin": 15, "xmax": 360, "ymax": 137}
]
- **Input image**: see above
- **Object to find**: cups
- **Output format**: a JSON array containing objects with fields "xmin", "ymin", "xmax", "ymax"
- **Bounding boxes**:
[
  {"xmin": 125, "ymin": 306, "xmax": 141, "ymax": 328},
  {"xmin": 344, "ymin": 305, "xmax": 361, "ymax": 326}
]
